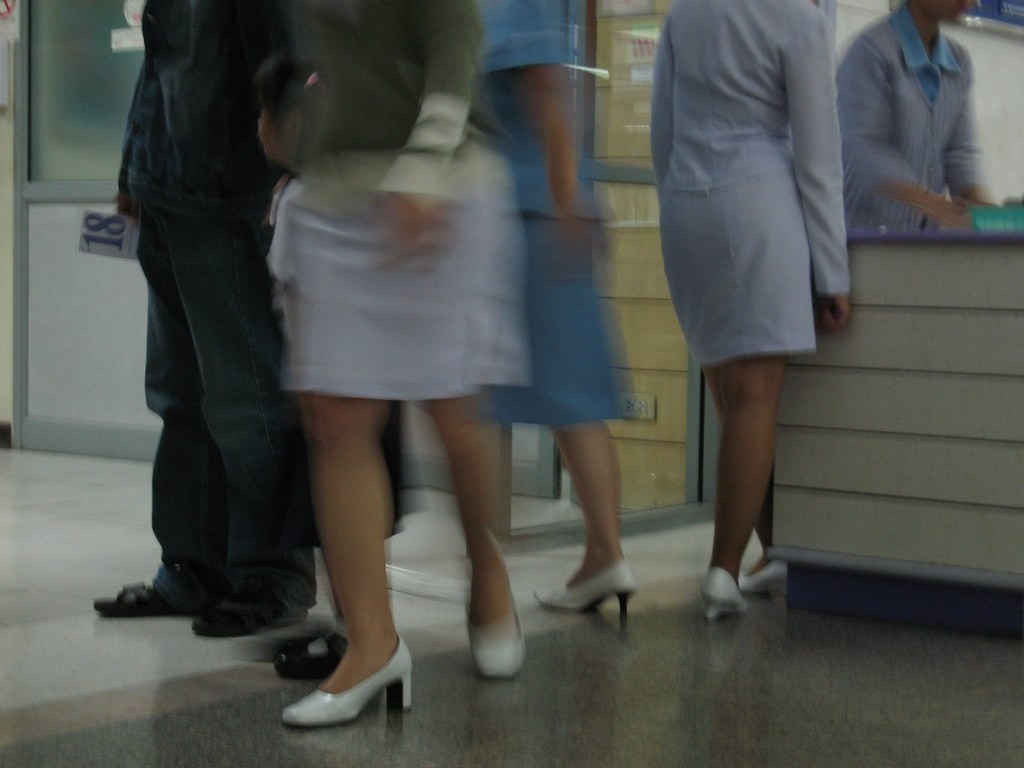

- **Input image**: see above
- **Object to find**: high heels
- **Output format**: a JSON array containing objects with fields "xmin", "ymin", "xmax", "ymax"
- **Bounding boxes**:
[
  {"xmin": 739, "ymin": 559, "xmax": 787, "ymax": 596},
  {"xmin": 281, "ymin": 632, "xmax": 411, "ymax": 726},
  {"xmin": 700, "ymin": 566, "xmax": 747, "ymax": 617},
  {"xmin": 466, "ymin": 597, "xmax": 525, "ymax": 680},
  {"xmin": 533, "ymin": 561, "xmax": 635, "ymax": 615}
]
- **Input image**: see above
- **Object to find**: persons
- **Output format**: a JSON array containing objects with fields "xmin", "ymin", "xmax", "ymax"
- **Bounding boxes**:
[
  {"xmin": 94, "ymin": 0, "xmax": 634, "ymax": 726},
  {"xmin": 650, "ymin": 0, "xmax": 852, "ymax": 620},
  {"xmin": 838, "ymin": 0, "xmax": 998, "ymax": 235}
]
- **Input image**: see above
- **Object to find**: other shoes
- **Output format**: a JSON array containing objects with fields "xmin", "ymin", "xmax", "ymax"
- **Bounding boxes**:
[
  {"xmin": 94, "ymin": 583, "xmax": 189, "ymax": 616},
  {"xmin": 192, "ymin": 596, "xmax": 310, "ymax": 635},
  {"xmin": 272, "ymin": 630, "xmax": 350, "ymax": 677}
]
